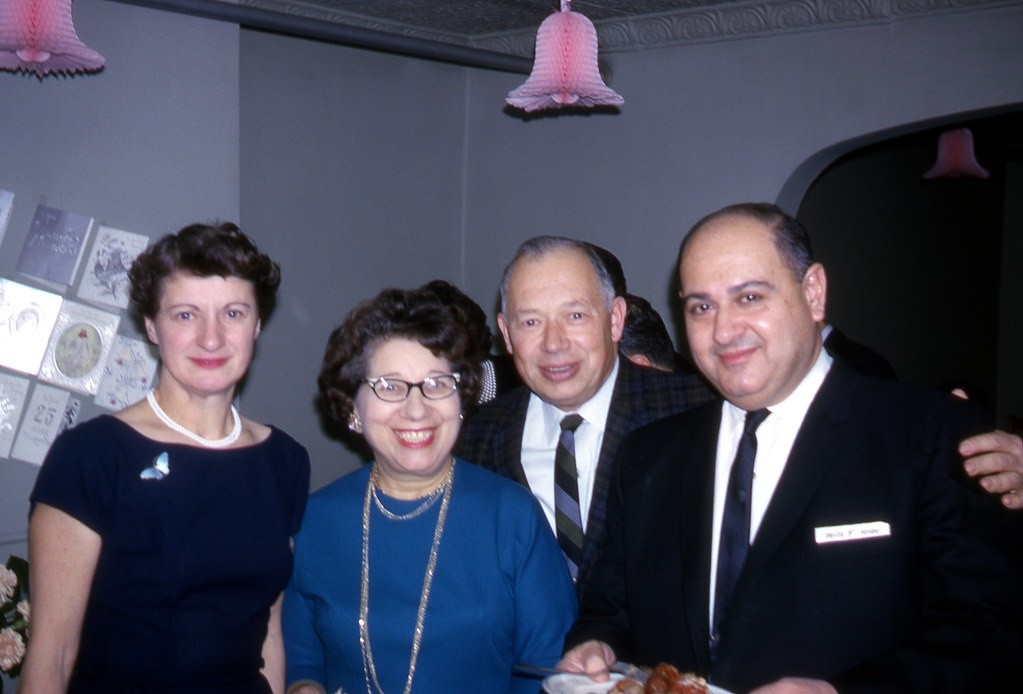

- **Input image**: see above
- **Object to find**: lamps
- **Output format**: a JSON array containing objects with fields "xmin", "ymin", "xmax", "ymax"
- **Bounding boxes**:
[
  {"xmin": 922, "ymin": 126, "xmax": 992, "ymax": 181},
  {"xmin": 505, "ymin": 0, "xmax": 625, "ymax": 112},
  {"xmin": 0, "ymin": 0, "xmax": 106, "ymax": 78}
]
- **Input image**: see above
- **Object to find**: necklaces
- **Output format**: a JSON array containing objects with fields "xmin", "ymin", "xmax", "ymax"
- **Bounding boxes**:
[
  {"xmin": 147, "ymin": 387, "xmax": 242, "ymax": 448},
  {"xmin": 359, "ymin": 456, "xmax": 455, "ymax": 694}
]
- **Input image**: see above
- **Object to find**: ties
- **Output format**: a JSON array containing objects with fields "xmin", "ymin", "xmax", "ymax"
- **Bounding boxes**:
[
  {"xmin": 555, "ymin": 414, "xmax": 584, "ymax": 585},
  {"xmin": 707, "ymin": 409, "xmax": 772, "ymax": 684}
]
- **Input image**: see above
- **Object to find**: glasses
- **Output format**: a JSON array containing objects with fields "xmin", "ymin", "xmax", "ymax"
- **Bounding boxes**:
[{"xmin": 362, "ymin": 373, "xmax": 460, "ymax": 402}]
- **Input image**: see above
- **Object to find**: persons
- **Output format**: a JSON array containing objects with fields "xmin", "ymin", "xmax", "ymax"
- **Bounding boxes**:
[
  {"xmin": 449, "ymin": 235, "xmax": 717, "ymax": 694},
  {"xmin": 17, "ymin": 221, "xmax": 311, "ymax": 694},
  {"xmin": 556, "ymin": 202, "xmax": 1023, "ymax": 694},
  {"xmin": 280, "ymin": 281, "xmax": 578, "ymax": 694}
]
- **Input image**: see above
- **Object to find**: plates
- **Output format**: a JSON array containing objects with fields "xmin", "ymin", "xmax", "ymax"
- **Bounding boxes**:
[{"xmin": 542, "ymin": 671, "xmax": 733, "ymax": 694}]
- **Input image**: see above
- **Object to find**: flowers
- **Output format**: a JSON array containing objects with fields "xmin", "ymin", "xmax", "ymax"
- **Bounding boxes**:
[{"xmin": 0, "ymin": 555, "xmax": 31, "ymax": 676}]
